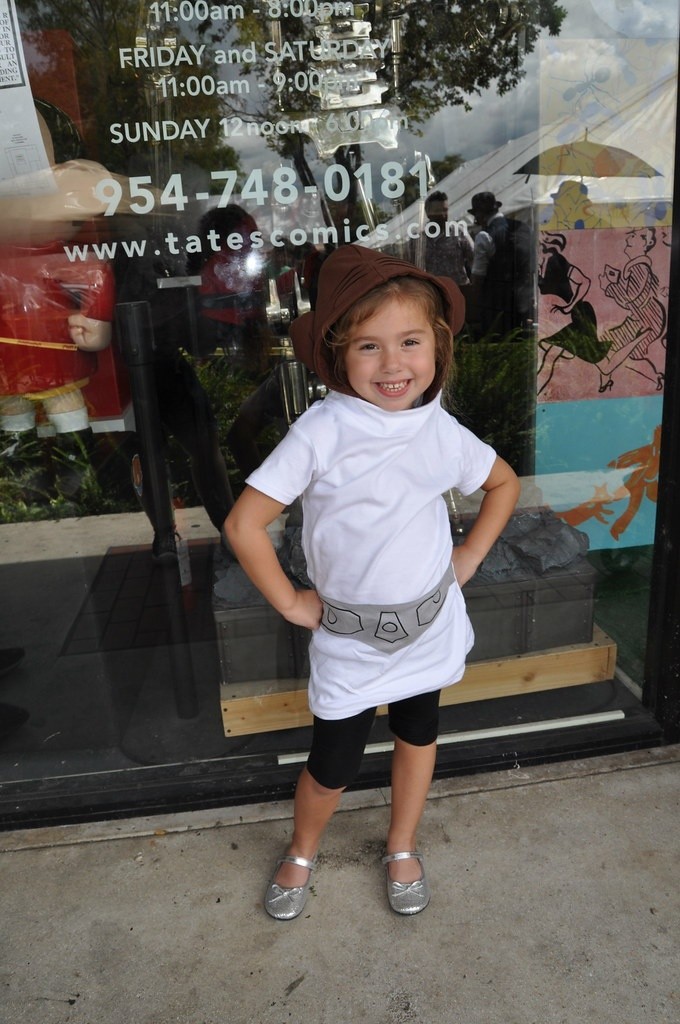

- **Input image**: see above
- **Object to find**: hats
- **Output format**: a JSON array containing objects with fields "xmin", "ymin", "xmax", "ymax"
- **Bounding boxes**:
[{"xmin": 289, "ymin": 243, "xmax": 464, "ymax": 402}]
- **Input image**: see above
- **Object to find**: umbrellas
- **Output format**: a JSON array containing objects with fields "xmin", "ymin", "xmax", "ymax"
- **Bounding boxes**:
[{"xmin": 511, "ymin": 125, "xmax": 663, "ymax": 185}]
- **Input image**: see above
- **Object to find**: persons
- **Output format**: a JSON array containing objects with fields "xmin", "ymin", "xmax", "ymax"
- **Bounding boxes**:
[
  {"xmin": 0, "ymin": 181, "xmax": 616, "ymax": 743},
  {"xmin": 224, "ymin": 250, "xmax": 519, "ymax": 921},
  {"xmin": 0, "ymin": 91, "xmax": 118, "ymax": 460}
]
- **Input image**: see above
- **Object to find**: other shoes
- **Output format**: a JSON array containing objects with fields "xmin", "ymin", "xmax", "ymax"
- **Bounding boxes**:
[
  {"xmin": 381, "ymin": 850, "xmax": 430, "ymax": 916},
  {"xmin": 264, "ymin": 855, "xmax": 318, "ymax": 920}
]
